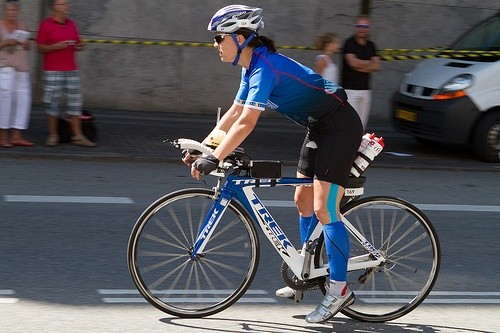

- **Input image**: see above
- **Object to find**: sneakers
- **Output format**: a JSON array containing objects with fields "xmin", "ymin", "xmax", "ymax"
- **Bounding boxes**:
[
  {"xmin": 305, "ymin": 285, "xmax": 356, "ymax": 323},
  {"xmin": 275, "ymin": 279, "xmax": 327, "ymax": 297}
]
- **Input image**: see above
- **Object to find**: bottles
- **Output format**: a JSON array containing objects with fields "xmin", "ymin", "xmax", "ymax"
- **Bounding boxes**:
[
  {"xmin": 350, "ymin": 137, "xmax": 384, "ymax": 178},
  {"xmin": 359, "ymin": 133, "xmax": 375, "ymax": 152}
]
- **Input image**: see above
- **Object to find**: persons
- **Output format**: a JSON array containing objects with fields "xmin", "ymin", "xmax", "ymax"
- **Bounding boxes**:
[
  {"xmin": 338, "ymin": 16, "xmax": 383, "ymax": 131},
  {"xmin": 35, "ymin": 0, "xmax": 96, "ymax": 147},
  {"xmin": 314, "ymin": 32, "xmax": 341, "ymax": 82},
  {"xmin": 0, "ymin": 0, "xmax": 33, "ymax": 147},
  {"xmin": 181, "ymin": 5, "xmax": 364, "ymax": 323}
]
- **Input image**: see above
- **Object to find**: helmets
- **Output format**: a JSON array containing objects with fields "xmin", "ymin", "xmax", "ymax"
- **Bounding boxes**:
[{"xmin": 207, "ymin": 4, "xmax": 264, "ymax": 33}]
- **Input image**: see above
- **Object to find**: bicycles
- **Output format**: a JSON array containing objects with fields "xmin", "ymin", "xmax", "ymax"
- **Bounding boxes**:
[{"xmin": 126, "ymin": 130, "xmax": 442, "ymax": 323}]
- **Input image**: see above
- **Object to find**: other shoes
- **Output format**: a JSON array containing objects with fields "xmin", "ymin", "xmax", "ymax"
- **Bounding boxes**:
[
  {"xmin": 10, "ymin": 139, "xmax": 35, "ymax": 147},
  {"xmin": 73, "ymin": 135, "xmax": 96, "ymax": 147},
  {"xmin": 45, "ymin": 135, "xmax": 58, "ymax": 147},
  {"xmin": 0, "ymin": 140, "xmax": 13, "ymax": 147}
]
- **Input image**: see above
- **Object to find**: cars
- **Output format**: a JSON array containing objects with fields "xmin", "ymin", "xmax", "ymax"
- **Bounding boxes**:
[{"xmin": 389, "ymin": 13, "xmax": 500, "ymax": 163}]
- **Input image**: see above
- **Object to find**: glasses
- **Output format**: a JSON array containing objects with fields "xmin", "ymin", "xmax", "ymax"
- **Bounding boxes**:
[
  {"xmin": 55, "ymin": 1, "xmax": 72, "ymax": 7},
  {"xmin": 213, "ymin": 33, "xmax": 238, "ymax": 44},
  {"xmin": 352, "ymin": 23, "xmax": 371, "ymax": 30}
]
